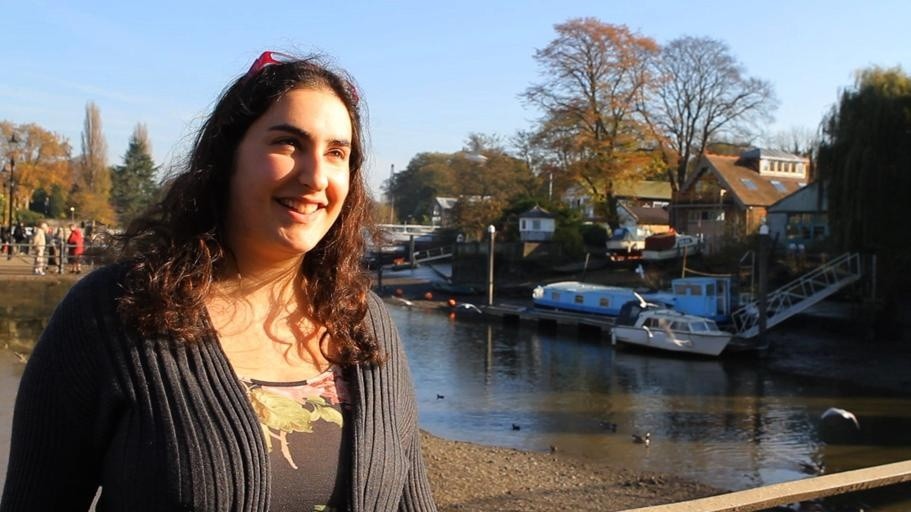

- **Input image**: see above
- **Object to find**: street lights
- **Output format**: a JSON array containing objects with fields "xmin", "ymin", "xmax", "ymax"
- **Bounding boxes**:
[
  {"xmin": 7, "ymin": 131, "xmax": 21, "ymax": 260},
  {"xmin": 70, "ymin": 206, "xmax": 75, "ymax": 222},
  {"xmin": 487, "ymin": 225, "xmax": 495, "ymax": 306}
]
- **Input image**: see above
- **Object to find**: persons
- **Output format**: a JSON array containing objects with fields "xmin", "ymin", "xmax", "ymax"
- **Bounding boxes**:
[
  {"xmin": 0, "ymin": 50, "xmax": 438, "ymax": 512},
  {"xmin": 0, "ymin": 222, "xmax": 94, "ymax": 276}
]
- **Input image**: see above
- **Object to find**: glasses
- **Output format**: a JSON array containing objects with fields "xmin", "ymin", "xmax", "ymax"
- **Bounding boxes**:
[{"xmin": 247, "ymin": 50, "xmax": 359, "ymax": 105}]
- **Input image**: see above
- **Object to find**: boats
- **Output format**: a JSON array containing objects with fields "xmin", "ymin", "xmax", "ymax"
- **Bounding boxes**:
[
  {"xmin": 640, "ymin": 234, "xmax": 705, "ymax": 261},
  {"xmin": 533, "ymin": 278, "xmax": 731, "ymax": 323},
  {"xmin": 611, "ymin": 308, "xmax": 733, "ymax": 357}
]
